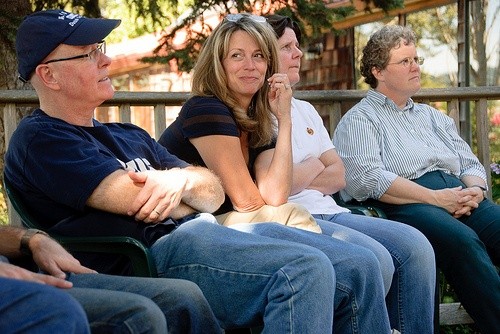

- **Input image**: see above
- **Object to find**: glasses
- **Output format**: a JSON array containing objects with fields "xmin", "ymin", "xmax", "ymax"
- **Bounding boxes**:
[
  {"xmin": 44, "ymin": 40, "xmax": 106, "ymax": 65},
  {"xmin": 382, "ymin": 57, "xmax": 425, "ymax": 67},
  {"xmin": 220, "ymin": 14, "xmax": 268, "ymax": 28}
]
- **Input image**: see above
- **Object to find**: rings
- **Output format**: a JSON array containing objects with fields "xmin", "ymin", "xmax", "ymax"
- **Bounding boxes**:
[
  {"xmin": 285, "ymin": 84, "xmax": 292, "ymax": 90},
  {"xmin": 153, "ymin": 209, "xmax": 161, "ymax": 215}
]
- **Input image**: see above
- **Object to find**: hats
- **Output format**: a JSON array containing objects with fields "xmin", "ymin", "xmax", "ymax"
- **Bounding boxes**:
[{"xmin": 16, "ymin": 10, "xmax": 121, "ymax": 83}]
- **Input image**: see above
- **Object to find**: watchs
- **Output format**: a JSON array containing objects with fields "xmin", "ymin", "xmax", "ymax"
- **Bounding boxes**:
[
  {"xmin": 20, "ymin": 228, "xmax": 50, "ymax": 274},
  {"xmin": 471, "ymin": 183, "xmax": 488, "ymax": 201}
]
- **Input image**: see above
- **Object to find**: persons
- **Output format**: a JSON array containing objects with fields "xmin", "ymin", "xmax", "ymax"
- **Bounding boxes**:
[
  {"xmin": 0, "ymin": 224, "xmax": 222, "ymax": 334},
  {"xmin": 5, "ymin": 11, "xmax": 395, "ymax": 334},
  {"xmin": 158, "ymin": 17, "xmax": 500, "ymax": 334}
]
